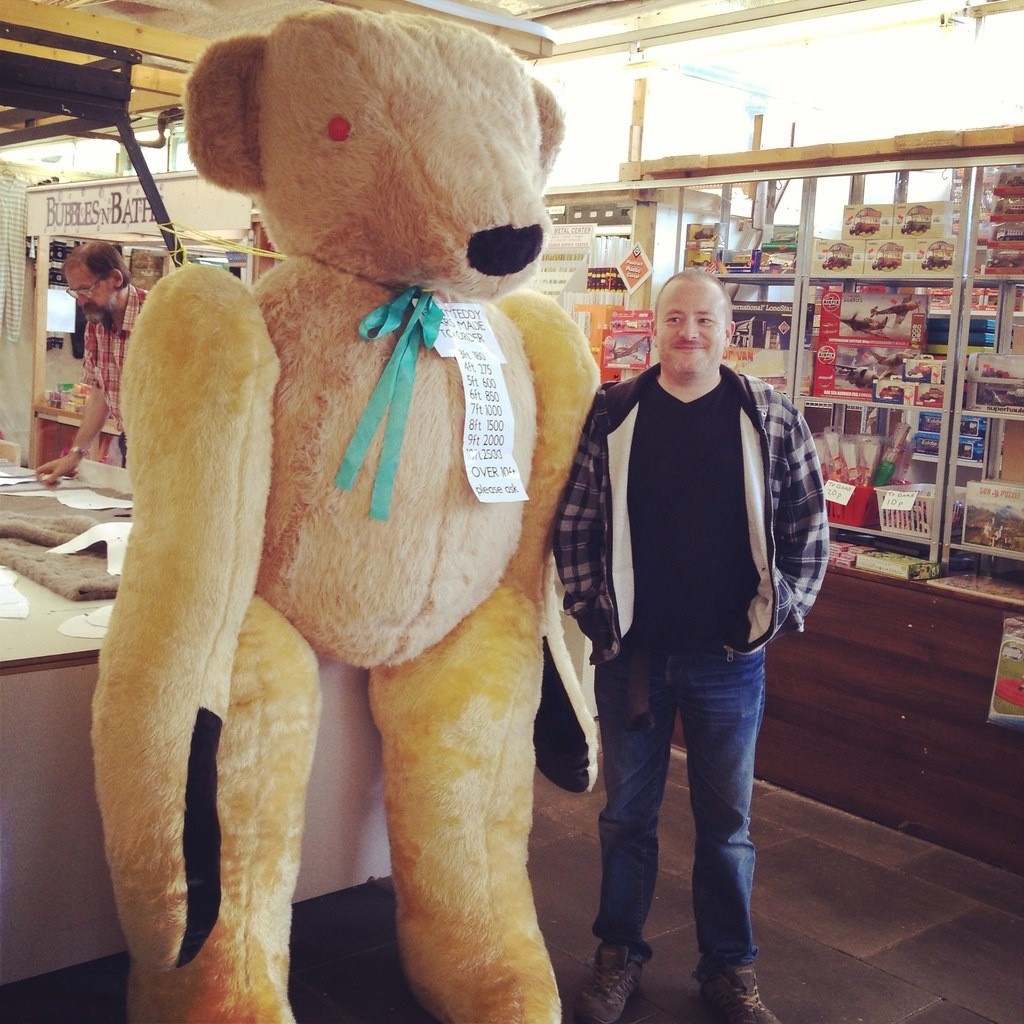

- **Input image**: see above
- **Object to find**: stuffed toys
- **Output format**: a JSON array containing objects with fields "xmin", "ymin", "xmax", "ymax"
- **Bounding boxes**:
[{"xmin": 88, "ymin": 5, "xmax": 601, "ymax": 1023}]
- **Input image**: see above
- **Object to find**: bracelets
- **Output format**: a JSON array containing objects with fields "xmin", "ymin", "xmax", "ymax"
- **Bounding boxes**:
[{"xmin": 69, "ymin": 447, "xmax": 85, "ymax": 456}]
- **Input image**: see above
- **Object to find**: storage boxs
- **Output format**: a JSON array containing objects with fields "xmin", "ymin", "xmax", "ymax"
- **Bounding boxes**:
[{"xmin": 683, "ymin": 201, "xmax": 1015, "ymax": 468}]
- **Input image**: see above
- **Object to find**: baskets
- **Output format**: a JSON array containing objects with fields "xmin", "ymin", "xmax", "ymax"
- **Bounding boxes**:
[
  {"xmin": 873, "ymin": 483, "xmax": 967, "ymax": 538},
  {"xmin": 825, "ymin": 479, "xmax": 912, "ymax": 527}
]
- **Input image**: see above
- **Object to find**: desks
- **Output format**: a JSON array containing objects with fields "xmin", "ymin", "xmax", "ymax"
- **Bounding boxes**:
[{"xmin": 0, "ymin": 476, "xmax": 392, "ymax": 982}]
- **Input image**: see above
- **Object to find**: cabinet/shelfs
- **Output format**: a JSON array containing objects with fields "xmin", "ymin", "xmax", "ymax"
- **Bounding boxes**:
[
  {"xmin": 29, "ymin": 213, "xmax": 274, "ymax": 468},
  {"xmin": 541, "ymin": 150, "xmax": 1024, "ymax": 874}
]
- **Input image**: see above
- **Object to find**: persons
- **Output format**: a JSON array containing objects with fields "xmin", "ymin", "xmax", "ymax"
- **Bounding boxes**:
[
  {"xmin": 555, "ymin": 271, "xmax": 830, "ymax": 1024},
  {"xmin": 37, "ymin": 242, "xmax": 149, "ymax": 486}
]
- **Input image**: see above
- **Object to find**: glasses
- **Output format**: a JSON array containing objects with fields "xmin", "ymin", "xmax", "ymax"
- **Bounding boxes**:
[{"xmin": 66, "ymin": 269, "xmax": 114, "ymax": 300}]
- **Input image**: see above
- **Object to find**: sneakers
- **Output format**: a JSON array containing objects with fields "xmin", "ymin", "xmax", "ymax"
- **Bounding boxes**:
[
  {"xmin": 572, "ymin": 941, "xmax": 652, "ymax": 1024},
  {"xmin": 695, "ymin": 952, "xmax": 783, "ymax": 1024}
]
